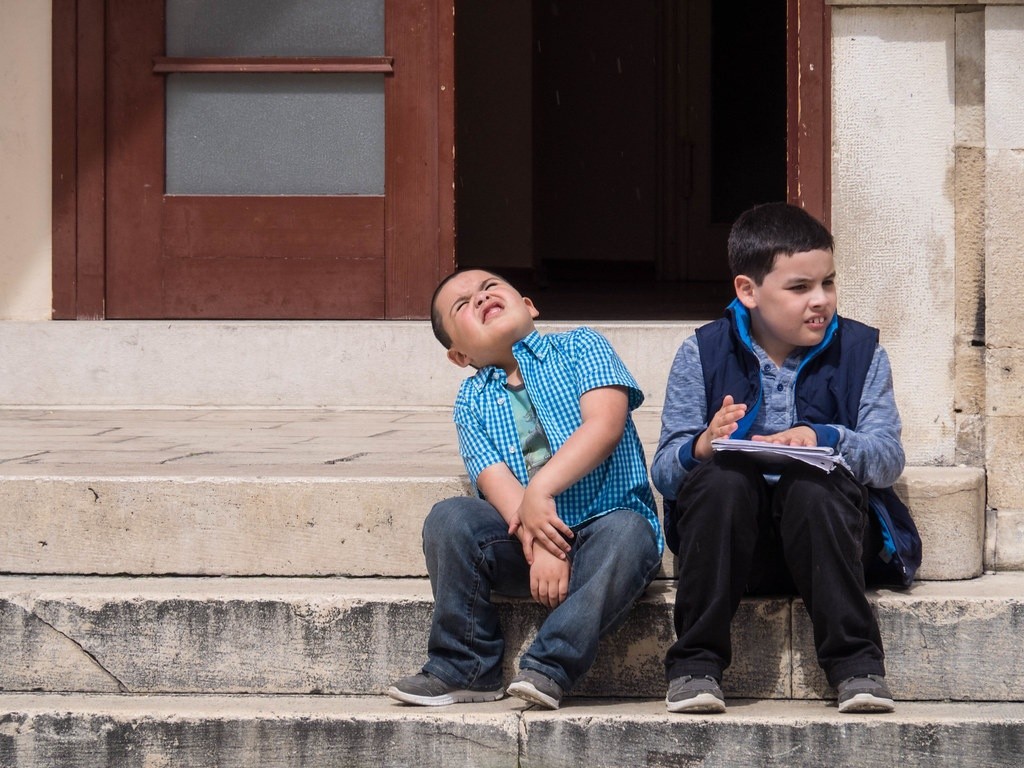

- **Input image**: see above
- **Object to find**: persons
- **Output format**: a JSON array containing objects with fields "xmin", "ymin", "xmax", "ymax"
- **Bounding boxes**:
[
  {"xmin": 651, "ymin": 203, "xmax": 922, "ymax": 711},
  {"xmin": 388, "ymin": 268, "xmax": 665, "ymax": 709}
]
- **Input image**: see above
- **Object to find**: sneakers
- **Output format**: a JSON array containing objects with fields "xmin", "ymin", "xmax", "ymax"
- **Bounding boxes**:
[
  {"xmin": 665, "ymin": 673, "xmax": 726, "ymax": 713},
  {"xmin": 835, "ymin": 673, "xmax": 894, "ymax": 714},
  {"xmin": 507, "ymin": 668, "xmax": 565, "ymax": 709},
  {"xmin": 389, "ymin": 669, "xmax": 503, "ymax": 705}
]
